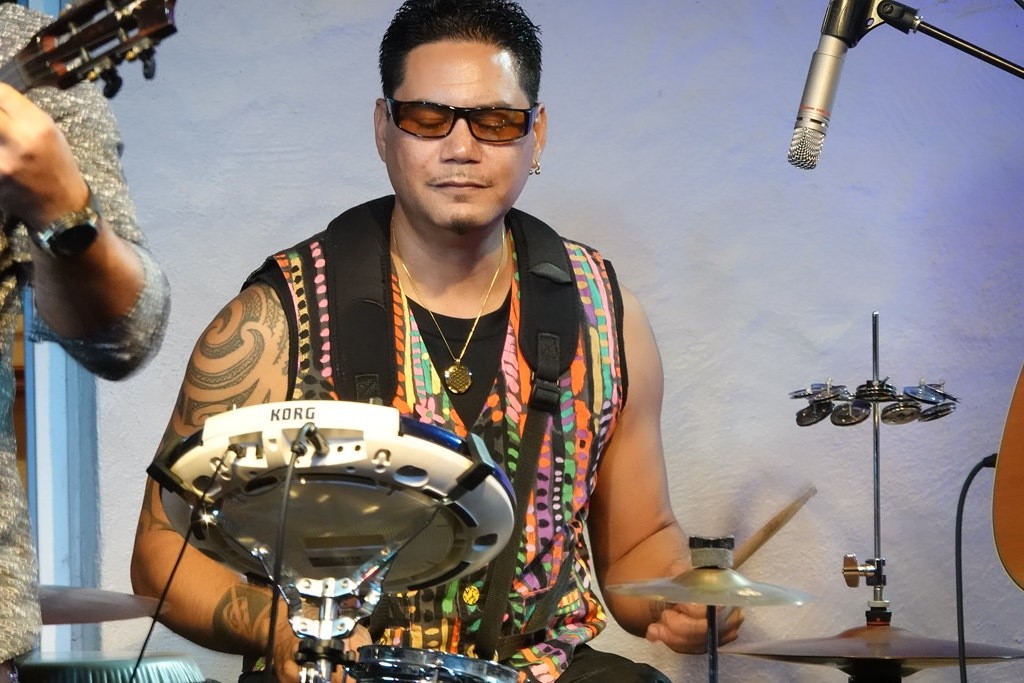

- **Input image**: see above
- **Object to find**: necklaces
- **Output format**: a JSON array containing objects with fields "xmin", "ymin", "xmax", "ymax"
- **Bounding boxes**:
[{"xmin": 392, "ymin": 211, "xmax": 506, "ymax": 393}]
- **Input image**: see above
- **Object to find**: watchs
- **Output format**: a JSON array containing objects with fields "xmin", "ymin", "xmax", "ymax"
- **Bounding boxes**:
[{"xmin": 22, "ymin": 177, "xmax": 103, "ymax": 261}]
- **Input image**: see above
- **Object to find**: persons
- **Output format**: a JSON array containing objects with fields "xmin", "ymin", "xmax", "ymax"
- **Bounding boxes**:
[
  {"xmin": 132, "ymin": 0, "xmax": 746, "ymax": 682},
  {"xmin": 1, "ymin": 1, "xmax": 174, "ymax": 683}
]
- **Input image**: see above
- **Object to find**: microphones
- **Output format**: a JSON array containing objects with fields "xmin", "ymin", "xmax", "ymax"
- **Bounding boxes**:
[{"xmin": 787, "ymin": 0, "xmax": 870, "ymax": 171}]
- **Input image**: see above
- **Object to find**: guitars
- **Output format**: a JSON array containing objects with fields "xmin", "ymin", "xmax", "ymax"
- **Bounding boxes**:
[{"xmin": 1, "ymin": 0, "xmax": 178, "ymax": 100}]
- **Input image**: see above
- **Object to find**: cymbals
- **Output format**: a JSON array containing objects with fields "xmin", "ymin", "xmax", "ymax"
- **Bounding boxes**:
[
  {"xmin": 788, "ymin": 376, "xmax": 961, "ymax": 428},
  {"xmin": 716, "ymin": 624, "xmax": 1024, "ymax": 679},
  {"xmin": 604, "ymin": 566, "xmax": 804, "ymax": 610}
]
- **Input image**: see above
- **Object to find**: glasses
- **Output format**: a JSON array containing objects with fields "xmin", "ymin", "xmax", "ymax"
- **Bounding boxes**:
[{"xmin": 384, "ymin": 95, "xmax": 540, "ymax": 143}]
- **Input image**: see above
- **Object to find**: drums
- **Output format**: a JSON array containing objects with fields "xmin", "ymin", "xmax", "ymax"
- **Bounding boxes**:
[
  {"xmin": 159, "ymin": 401, "xmax": 517, "ymax": 600},
  {"xmin": 353, "ymin": 645, "xmax": 521, "ymax": 683}
]
